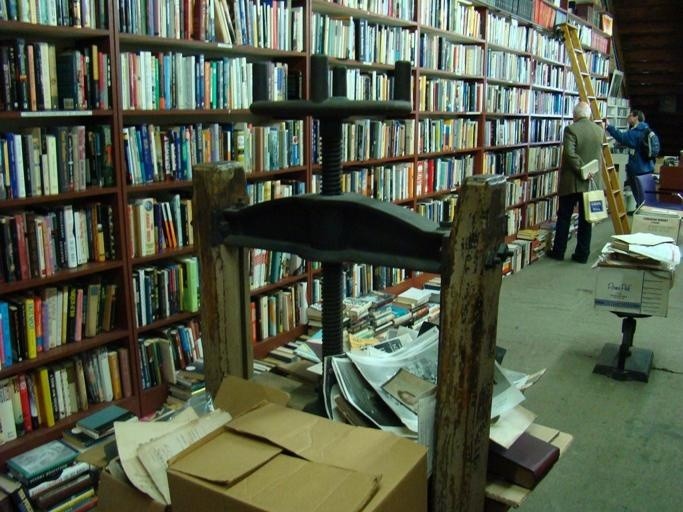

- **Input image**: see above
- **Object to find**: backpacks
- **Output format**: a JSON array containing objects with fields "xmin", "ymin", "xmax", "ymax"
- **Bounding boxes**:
[{"xmin": 631, "ymin": 127, "xmax": 659, "ymax": 158}]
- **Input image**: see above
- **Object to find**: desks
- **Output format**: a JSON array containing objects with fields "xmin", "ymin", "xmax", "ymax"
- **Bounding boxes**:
[{"xmin": 660, "ymin": 151, "xmax": 683, "ymax": 203}]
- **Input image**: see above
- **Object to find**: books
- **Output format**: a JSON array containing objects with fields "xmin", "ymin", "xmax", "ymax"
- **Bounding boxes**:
[{"xmin": 1, "ymin": 1, "xmax": 633, "ymax": 512}]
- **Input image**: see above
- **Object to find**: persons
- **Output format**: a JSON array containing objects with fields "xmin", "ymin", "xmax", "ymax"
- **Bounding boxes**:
[{"xmin": 606, "ymin": 109, "xmax": 656, "ymax": 203}]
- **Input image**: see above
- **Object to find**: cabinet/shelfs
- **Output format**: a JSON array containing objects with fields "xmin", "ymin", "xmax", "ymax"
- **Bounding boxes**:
[{"xmin": 0, "ymin": 1, "xmax": 635, "ymax": 476}]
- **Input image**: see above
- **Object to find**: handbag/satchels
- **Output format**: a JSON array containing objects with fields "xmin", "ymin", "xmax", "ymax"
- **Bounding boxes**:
[{"xmin": 582, "ymin": 178, "xmax": 608, "ymax": 223}]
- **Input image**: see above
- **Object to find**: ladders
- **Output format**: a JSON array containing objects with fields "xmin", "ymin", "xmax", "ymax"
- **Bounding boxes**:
[{"xmin": 558, "ymin": 22, "xmax": 630, "ymax": 236}]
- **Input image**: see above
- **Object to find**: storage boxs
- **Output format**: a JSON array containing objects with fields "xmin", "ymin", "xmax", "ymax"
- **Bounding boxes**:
[
  {"xmin": 165, "ymin": 398, "xmax": 429, "ymax": 511},
  {"xmin": 591, "ymin": 201, "xmax": 683, "ymax": 317}
]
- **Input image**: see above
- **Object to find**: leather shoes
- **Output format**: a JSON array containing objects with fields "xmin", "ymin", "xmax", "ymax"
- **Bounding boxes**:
[
  {"xmin": 571, "ymin": 253, "xmax": 587, "ymax": 263},
  {"xmin": 545, "ymin": 250, "xmax": 564, "ymax": 261}
]
